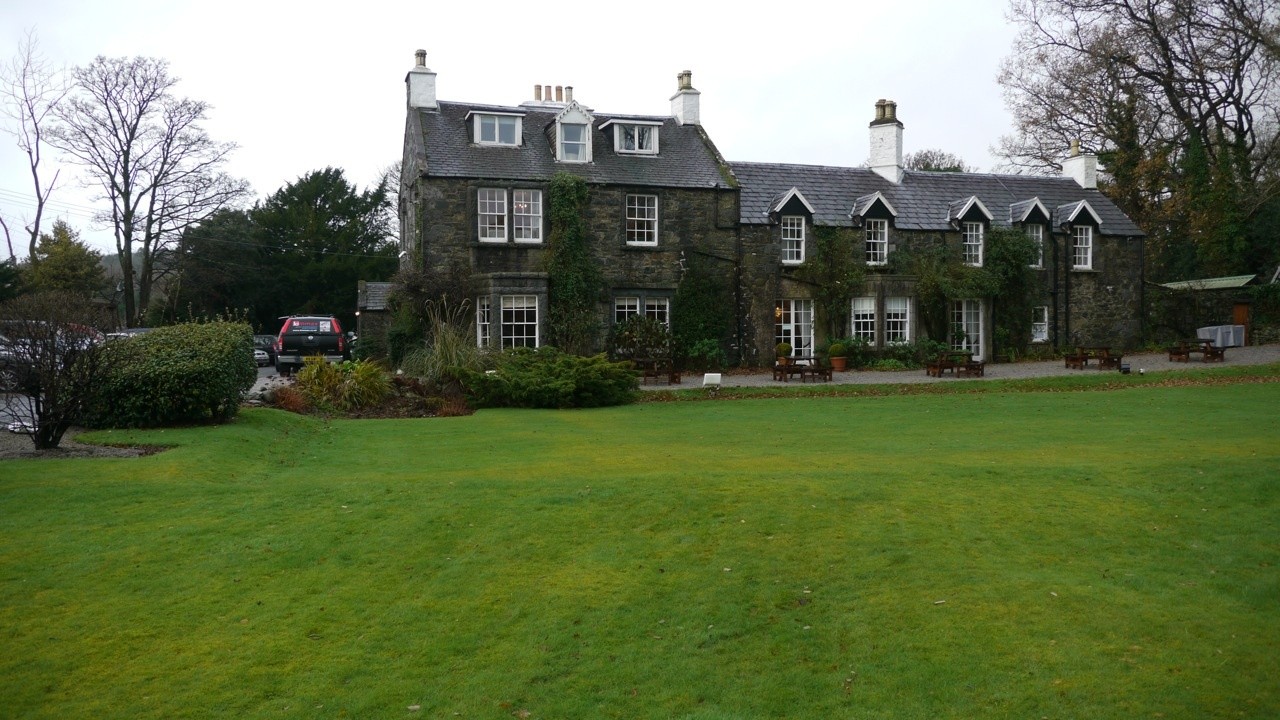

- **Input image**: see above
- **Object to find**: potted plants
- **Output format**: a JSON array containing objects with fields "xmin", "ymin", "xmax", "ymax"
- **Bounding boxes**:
[
  {"xmin": 829, "ymin": 344, "xmax": 848, "ymax": 372},
  {"xmin": 775, "ymin": 343, "xmax": 792, "ymax": 364}
]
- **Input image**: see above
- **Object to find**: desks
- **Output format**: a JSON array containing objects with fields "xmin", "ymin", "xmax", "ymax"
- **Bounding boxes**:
[
  {"xmin": 1072, "ymin": 346, "xmax": 1113, "ymax": 369},
  {"xmin": 779, "ymin": 356, "xmax": 828, "ymax": 382},
  {"xmin": 637, "ymin": 359, "xmax": 673, "ymax": 385},
  {"xmin": 1175, "ymin": 339, "xmax": 1217, "ymax": 361},
  {"xmin": 932, "ymin": 352, "xmax": 979, "ymax": 378}
]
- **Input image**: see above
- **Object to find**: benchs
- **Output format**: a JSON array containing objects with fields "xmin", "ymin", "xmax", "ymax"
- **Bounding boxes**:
[
  {"xmin": 1166, "ymin": 346, "xmax": 1225, "ymax": 364},
  {"xmin": 773, "ymin": 364, "xmax": 835, "ymax": 383},
  {"xmin": 926, "ymin": 360, "xmax": 985, "ymax": 378},
  {"xmin": 636, "ymin": 369, "xmax": 681, "ymax": 386},
  {"xmin": 1065, "ymin": 352, "xmax": 1124, "ymax": 370}
]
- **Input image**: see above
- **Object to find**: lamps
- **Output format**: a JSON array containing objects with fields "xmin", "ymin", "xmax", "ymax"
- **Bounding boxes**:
[
  {"xmin": 1121, "ymin": 364, "xmax": 1131, "ymax": 374},
  {"xmin": 703, "ymin": 373, "xmax": 721, "ymax": 390}
]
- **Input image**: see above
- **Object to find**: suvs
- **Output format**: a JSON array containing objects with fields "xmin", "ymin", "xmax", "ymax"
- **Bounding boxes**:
[{"xmin": 276, "ymin": 315, "xmax": 351, "ymax": 373}]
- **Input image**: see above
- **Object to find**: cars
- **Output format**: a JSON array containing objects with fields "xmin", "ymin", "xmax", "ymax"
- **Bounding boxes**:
[
  {"xmin": 0, "ymin": 320, "xmax": 155, "ymax": 391},
  {"xmin": 253, "ymin": 335, "xmax": 278, "ymax": 366}
]
